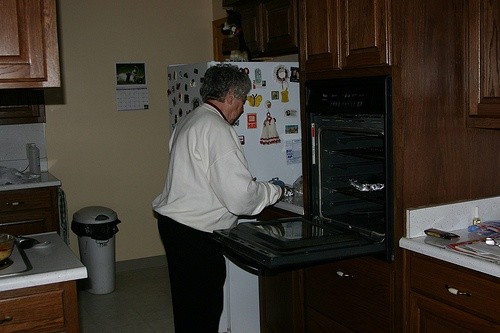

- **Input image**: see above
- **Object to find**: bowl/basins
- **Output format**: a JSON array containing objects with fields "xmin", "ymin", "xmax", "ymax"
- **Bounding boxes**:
[{"xmin": 0, "ymin": 234, "xmax": 15, "ymax": 262}]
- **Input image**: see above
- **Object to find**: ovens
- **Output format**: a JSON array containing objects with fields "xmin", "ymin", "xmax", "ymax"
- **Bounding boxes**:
[{"xmin": 209, "ymin": 76, "xmax": 391, "ymax": 269}]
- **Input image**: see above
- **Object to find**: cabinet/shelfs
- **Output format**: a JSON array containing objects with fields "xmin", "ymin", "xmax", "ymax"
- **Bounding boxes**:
[
  {"xmin": 0, "ymin": 0, "xmax": 61, "ymax": 88},
  {"xmin": 0, "ymin": 181, "xmax": 87, "ymax": 333},
  {"xmin": 213, "ymin": 1, "xmax": 500, "ymax": 333}
]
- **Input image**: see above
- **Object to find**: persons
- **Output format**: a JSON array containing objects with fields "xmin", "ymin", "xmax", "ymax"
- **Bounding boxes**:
[
  {"xmin": 126, "ymin": 67, "xmax": 139, "ymax": 84},
  {"xmin": 151, "ymin": 64, "xmax": 285, "ymax": 333}
]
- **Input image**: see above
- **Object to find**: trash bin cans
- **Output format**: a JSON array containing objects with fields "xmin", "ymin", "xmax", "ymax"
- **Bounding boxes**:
[{"xmin": 71, "ymin": 206, "xmax": 122, "ymax": 295}]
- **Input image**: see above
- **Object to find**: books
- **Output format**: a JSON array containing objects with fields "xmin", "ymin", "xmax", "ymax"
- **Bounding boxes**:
[{"xmin": 446, "ymin": 235, "xmax": 500, "ymax": 265}]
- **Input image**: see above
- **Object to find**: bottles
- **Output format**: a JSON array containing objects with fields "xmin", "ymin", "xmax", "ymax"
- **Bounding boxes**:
[{"xmin": 29, "ymin": 143, "xmax": 41, "ymax": 175}]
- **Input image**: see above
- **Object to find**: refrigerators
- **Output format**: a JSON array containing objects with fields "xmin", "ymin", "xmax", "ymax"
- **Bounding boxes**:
[{"xmin": 167, "ymin": 61, "xmax": 299, "ymax": 333}]
